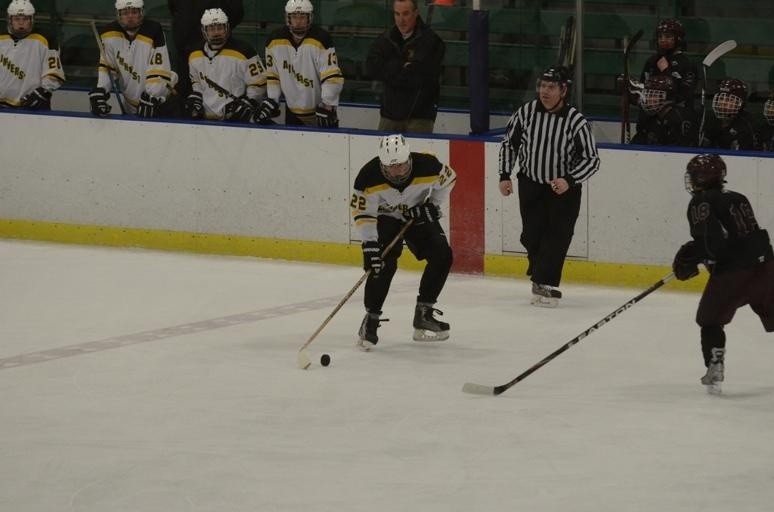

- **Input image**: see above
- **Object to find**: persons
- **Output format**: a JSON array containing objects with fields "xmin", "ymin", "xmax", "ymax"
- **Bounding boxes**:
[
  {"xmin": 249, "ymin": 0, "xmax": 344, "ymax": 130},
  {"xmin": 350, "ymin": 133, "xmax": 457, "ymax": 345},
  {"xmin": 365, "ymin": 1, "xmax": 446, "ymax": 134},
  {"xmin": 88, "ymin": 0, "xmax": 183, "ymax": 120},
  {"xmin": 497, "ymin": 63, "xmax": 600, "ymax": 299},
  {"xmin": 672, "ymin": 154, "xmax": 773, "ymax": 385},
  {"xmin": 185, "ymin": 8, "xmax": 267, "ymax": 123},
  {"xmin": 617, "ymin": 17, "xmax": 774, "ymax": 153},
  {"xmin": 0, "ymin": 0, "xmax": 66, "ymax": 111}
]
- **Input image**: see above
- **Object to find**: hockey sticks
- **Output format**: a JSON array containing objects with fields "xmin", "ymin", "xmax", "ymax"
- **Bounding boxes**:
[
  {"xmin": 461, "ymin": 274, "xmax": 675, "ymax": 396},
  {"xmin": 623, "ymin": 29, "xmax": 644, "ymax": 143},
  {"xmin": 294, "ymin": 197, "xmax": 427, "ymax": 371},
  {"xmin": 697, "ymin": 39, "xmax": 738, "ymax": 148}
]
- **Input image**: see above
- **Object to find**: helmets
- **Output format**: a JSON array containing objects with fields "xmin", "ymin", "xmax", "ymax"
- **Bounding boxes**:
[
  {"xmin": 285, "ymin": 1, "xmax": 313, "ymax": 40},
  {"xmin": 685, "ymin": 154, "xmax": 726, "ymax": 198},
  {"xmin": 760, "ymin": 91, "xmax": 774, "ymax": 127},
  {"xmin": 200, "ymin": 8, "xmax": 230, "ymax": 50},
  {"xmin": 6, "ymin": 1, "xmax": 36, "ymax": 40},
  {"xmin": 535, "ymin": 65, "xmax": 569, "ymax": 95},
  {"xmin": 656, "ymin": 18, "xmax": 685, "ymax": 49},
  {"xmin": 639, "ymin": 76, "xmax": 675, "ymax": 117},
  {"xmin": 712, "ymin": 79, "xmax": 749, "ymax": 121},
  {"xmin": 379, "ymin": 134, "xmax": 413, "ymax": 188},
  {"xmin": 114, "ymin": 1, "xmax": 145, "ymax": 36}
]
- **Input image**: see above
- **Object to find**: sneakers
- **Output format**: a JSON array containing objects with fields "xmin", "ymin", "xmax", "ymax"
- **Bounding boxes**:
[
  {"xmin": 701, "ymin": 348, "xmax": 726, "ymax": 386},
  {"xmin": 358, "ymin": 314, "xmax": 389, "ymax": 346},
  {"xmin": 413, "ymin": 305, "xmax": 449, "ymax": 332},
  {"xmin": 532, "ymin": 284, "xmax": 561, "ymax": 299}
]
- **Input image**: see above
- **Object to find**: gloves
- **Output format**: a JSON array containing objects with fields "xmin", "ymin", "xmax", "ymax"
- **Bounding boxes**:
[
  {"xmin": 314, "ymin": 104, "xmax": 339, "ymax": 130},
  {"xmin": 224, "ymin": 96, "xmax": 259, "ymax": 122},
  {"xmin": 614, "ymin": 73, "xmax": 644, "ymax": 106},
  {"xmin": 21, "ymin": 87, "xmax": 52, "ymax": 111},
  {"xmin": 184, "ymin": 92, "xmax": 204, "ymax": 121},
  {"xmin": 361, "ymin": 241, "xmax": 386, "ymax": 282},
  {"xmin": 402, "ymin": 203, "xmax": 440, "ymax": 229},
  {"xmin": 253, "ymin": 99, "xmax": 281, "ymax": 126},
  {"xmin": 672, "ymin": 240, "xmax": 706, "ymax": 281},
  {"xmin": 88, "ymin": 88, "xmax": 112, "ymax": 114},
  {"xmin": 137, "ymin": 93, "xmax": 162, "ymax": 119}
]
